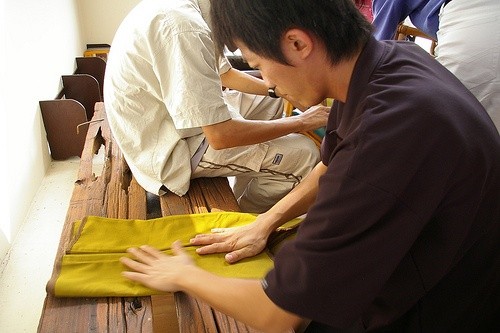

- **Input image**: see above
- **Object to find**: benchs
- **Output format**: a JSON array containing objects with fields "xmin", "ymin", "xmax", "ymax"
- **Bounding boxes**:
[{"xmin": 33, "ymin": 99, "xmax": 296, "ymax": 333}]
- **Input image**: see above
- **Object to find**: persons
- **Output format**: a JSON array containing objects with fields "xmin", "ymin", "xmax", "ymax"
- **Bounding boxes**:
[
  {"xmin": 368, "ymin": 0, "xmax": 500, "ymax": 135},
  {"xmin": 102, "ymin": 0, "xmax": 333, "ymax": 213},
  {"xmin": 118, "ymin": 1, "xmax": 500, "ymax": 333}
]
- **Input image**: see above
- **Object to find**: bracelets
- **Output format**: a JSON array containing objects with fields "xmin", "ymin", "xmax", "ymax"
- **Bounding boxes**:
[{"xmin": 268, "ymin": 84, "xmax": 281, "ymax": 99}]
